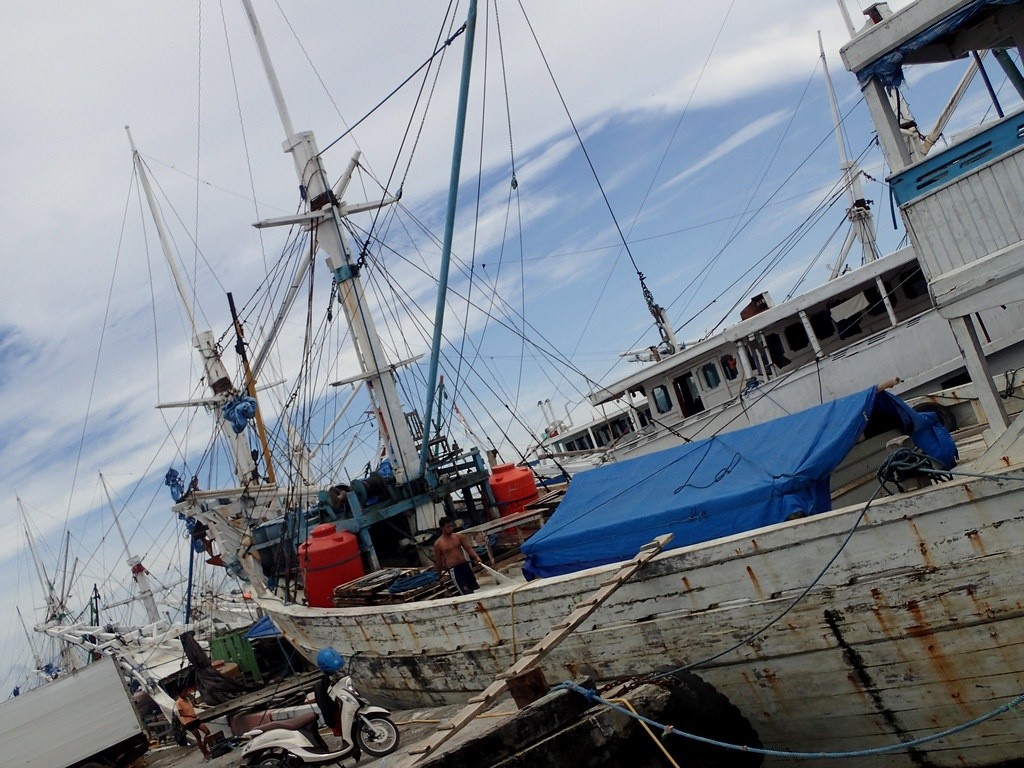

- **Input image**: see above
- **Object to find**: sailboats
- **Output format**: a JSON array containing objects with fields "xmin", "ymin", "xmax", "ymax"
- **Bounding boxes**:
[{"xmin": 11, "ymin": 0, "xmax": 1024, "ymax": 768}]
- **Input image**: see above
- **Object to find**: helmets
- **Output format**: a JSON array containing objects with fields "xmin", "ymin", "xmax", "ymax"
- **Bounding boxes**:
[{"xmin": 318, "ymin": 648, "xmax": 344, "ymax": 672}]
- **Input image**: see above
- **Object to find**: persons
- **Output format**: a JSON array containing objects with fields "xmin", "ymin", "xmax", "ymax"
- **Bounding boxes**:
[
  {"xmin": 434, "ymin": 517, "xmax": 481, "ymax": 596},
  {"xmin": 176, "ymin": 684, "xmax": 210, "ymax": 760}
]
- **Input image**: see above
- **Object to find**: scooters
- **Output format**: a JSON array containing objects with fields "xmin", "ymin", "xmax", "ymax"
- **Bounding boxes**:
[{"xmin": 231, "ymin": 648, "xmax": 399, "ymax": 768}]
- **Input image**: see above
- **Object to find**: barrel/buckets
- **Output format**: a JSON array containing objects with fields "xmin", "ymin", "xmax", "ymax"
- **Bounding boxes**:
[
  {"xmin": 211, "ymin": 660, "xmax": 243, "ymax": 683},
  {"xmin": 506, "ymin": 666, "xmax": 551, "ymax": 710},
  {"xmin": 297, "ymin": 521, "xmax": 366, "ymax": 607},
  {"xmin": 488, "ymin": 460, "xmax": 544, "ymax": 542},
  {"xmin": 251, "ymin": 506, "xmax": 319, "ymax": 569}
]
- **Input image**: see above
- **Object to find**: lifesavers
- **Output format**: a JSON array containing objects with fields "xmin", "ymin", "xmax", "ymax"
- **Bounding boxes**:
[
  {"xmin": 912, "ymin": 401, "xmax": 958, "ymax": 434},
  {"xmin": 649, "ymin": 664, "xmax": 766, "ymax": 768}
]
